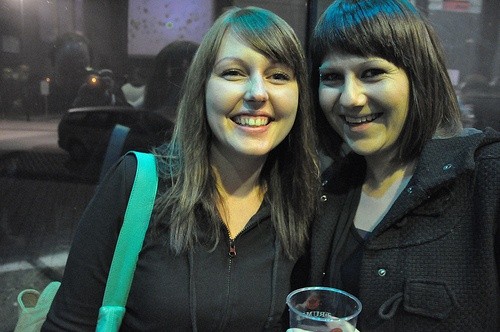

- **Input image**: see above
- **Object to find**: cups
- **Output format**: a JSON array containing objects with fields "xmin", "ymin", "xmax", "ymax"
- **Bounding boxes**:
[{"xmin": 286, "ymin": 286, "xmax": 362, "ymax": 332}]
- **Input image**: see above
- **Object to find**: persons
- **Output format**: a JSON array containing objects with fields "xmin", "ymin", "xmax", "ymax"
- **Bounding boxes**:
[
  {"xmin": 39, "ymin": 5, "xmax": 321, "ymax": 331},
  {"xmin": 141, "ymin": 40, "xmax": 201, "ymax": 123},
  {"xmin": 282, "ymin": 0, "xmax": 500, "ymax": 332}
]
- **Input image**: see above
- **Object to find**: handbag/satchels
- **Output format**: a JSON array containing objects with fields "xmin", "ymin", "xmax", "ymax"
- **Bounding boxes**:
[{"xmin": 11, "ymin": 149, "xmax": 160, "ymax": 332}]
